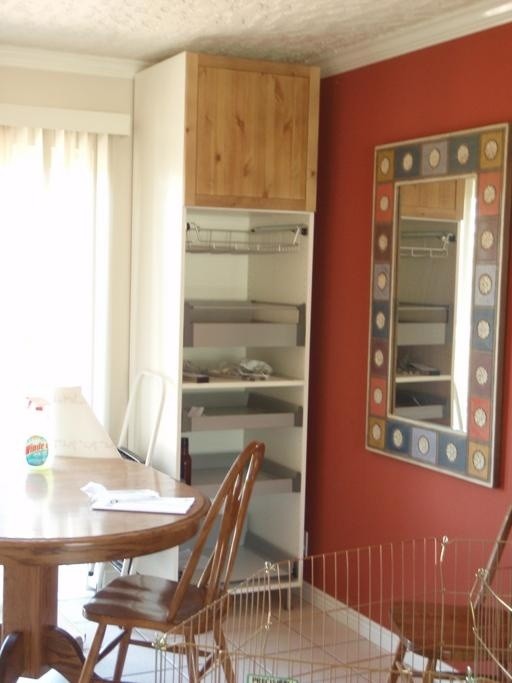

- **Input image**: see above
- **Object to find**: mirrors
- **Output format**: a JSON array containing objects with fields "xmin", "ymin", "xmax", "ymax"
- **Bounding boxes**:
[{"xmin": 365, "ymin": 120, "xmax": 505, "ymax": 490}]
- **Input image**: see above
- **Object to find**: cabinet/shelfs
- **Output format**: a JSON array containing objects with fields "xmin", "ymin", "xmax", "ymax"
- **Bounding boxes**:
[
  {"xmin": 129, "ymin": 50, "xmax": 320, "ymax": 600},
  {"xmin": 388, "ymin": 176, "xmax": 467, "ymax": 432}
]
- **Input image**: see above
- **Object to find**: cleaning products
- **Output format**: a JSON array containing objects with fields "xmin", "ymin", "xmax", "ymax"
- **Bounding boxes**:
[{"xmin": 22, "ymin": 395, "xmax": 55, "ymax": 473}]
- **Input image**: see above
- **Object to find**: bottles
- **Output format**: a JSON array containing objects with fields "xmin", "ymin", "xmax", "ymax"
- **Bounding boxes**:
[{"xmin": 180, "ymin": 436, "xmax": 192, "ymax": 485}]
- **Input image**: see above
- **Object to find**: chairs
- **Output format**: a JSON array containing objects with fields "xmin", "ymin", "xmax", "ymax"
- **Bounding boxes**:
[
  {"xmin": 87, "ymin": 371, "xmax": 166, "ymax": 593},
  {"xmin": 79, "ymin": 441, "xmax": 266, "ymax": 683},
  {"xmin": 387, "ymin": 505, "xmax": 512, "ymax": 683}
]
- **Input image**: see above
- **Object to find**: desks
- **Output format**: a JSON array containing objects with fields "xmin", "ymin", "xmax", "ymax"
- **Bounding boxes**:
[{"xmin": 1, "ymin": 454, "xmax": 210, "ymax": 683}]
